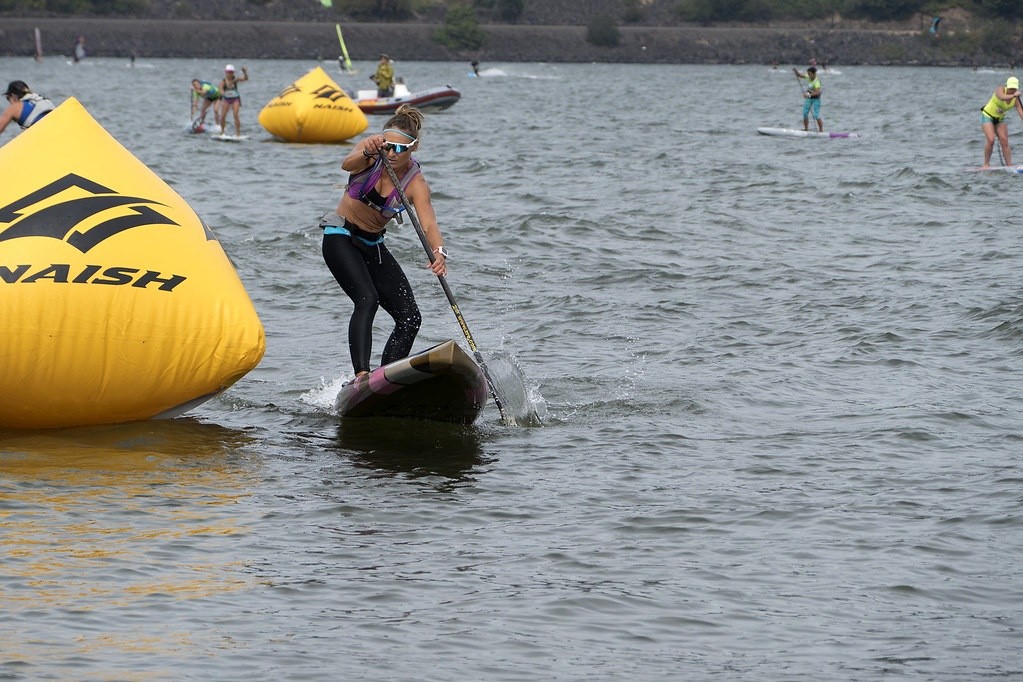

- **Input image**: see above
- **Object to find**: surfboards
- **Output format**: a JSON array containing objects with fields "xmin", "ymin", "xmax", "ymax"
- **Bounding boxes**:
[
  {"xmin": 978, "ymin": 164, "xmax": 1023, "ymax": 173},
  {"xmin": 337, "ymin": 340, "xmax": 488, "ymax": 426},
  {"xmin": 757, "ymin": 127, "xmax": 859, "ymax": 138},
  {"xmin": 185, "ymin": 122, "xmax": 248, "ymax": 141}
]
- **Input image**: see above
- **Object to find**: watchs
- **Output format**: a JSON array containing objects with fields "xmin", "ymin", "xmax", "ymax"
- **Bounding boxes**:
[{"xmin": 433, "ymin": 246, "xmax": 447, "ymax": 259}]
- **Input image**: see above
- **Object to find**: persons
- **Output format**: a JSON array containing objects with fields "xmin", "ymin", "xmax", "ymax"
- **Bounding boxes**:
[
  {"xmin": 794, "ymin": 67, "xmax": 824, "ymax": 133},
  {"xmin": 981, "ymin": 76, "xmax": 1023, "ymax": 169},
  {"xmin": 192, "ymin": 79, "xmax": 222, "ymax": 124},
  {"xmin": 374, "ymin": 53, "xmax": 395, "ymax": 98},
  {"xmin": 218, "ymin": 63, "xmax": 248, "ymax": 136},
  {"xmin": 318, "ymin": 103, "xmax": 448, "ymax": 378},
  {"xmin": 472, "ymin": 55, "xmax": 481, "ymax": 78},
  {"xmin": 0, "ymin": 80, "xmax": 56, "ymax": 137}
]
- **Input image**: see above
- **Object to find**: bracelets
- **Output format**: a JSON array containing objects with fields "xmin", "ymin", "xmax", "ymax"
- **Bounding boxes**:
[
  {"xmin": 809, "ymin": 94, "xmax": 811, "ymax": 98},
  {"xmin": 362, "ymin": 150, "xmax": 379, "ymax": 161}
]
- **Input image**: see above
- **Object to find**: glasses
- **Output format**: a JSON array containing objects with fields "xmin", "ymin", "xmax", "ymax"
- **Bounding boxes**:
[{"xmin": 384, "ymin": 139, "xmax": 416, "ymax": 153}]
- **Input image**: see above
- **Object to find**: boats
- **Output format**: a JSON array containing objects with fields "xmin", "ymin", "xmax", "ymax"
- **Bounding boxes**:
[{"xmin": 351, "ymin": 77, "xmax": 461, "ymax": 114}]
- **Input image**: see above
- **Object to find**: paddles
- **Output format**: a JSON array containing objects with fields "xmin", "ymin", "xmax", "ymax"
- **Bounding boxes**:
[
  {"xmin": 190, "ymin": 88, "xmax": 193, "ymax": 124},
  {"xmin": 193, "ymin": 72, "xmax": 245, "ymax": 127},
  {"xmin": 793, "ymin": 68, "xmax": 818, "ymax": 133},
  {"xmin": 378, "ymin": 147, "xmax": 509, "ymax": 423},
  {"xmin": 1014, "ymin": 95, "xmax": 1023, "ymax": 109}
]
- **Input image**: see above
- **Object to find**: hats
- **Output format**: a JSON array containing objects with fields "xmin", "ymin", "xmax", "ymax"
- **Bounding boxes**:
[
  {"xmin": 224, "ymin": 65, "xmax": 235, "ymax": 72},
  {"xmin": 1, "ymin": 81, "xmax": 30, "ymax": 99},
  {"xmin": 380, "ymin": 54, "xmax": 390, "ymax": 62},
  {"xmin": 1007, "ymin": 77, "xmax": 1019, "ymax": 89}
]
